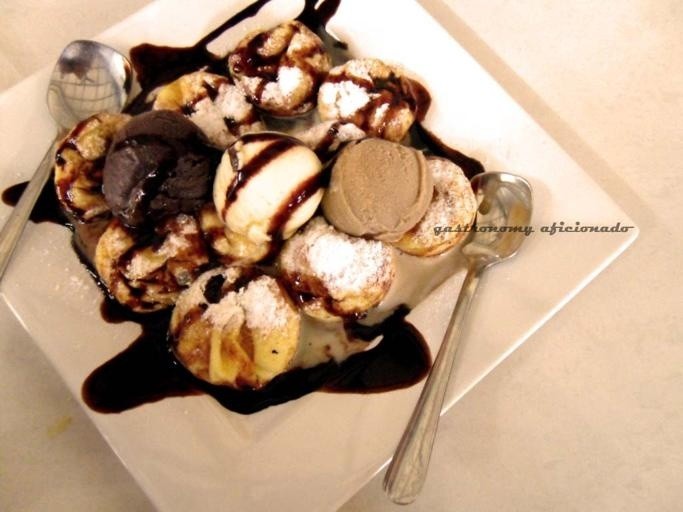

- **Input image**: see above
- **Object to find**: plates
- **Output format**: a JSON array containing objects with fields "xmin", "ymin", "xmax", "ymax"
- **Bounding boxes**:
[{"xmin": 2, "ymin": 1, "xmax": 640, "ymax": 512}]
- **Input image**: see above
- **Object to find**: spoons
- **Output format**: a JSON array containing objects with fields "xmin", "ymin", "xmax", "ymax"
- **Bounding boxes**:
[
  {"xmin": 383, "ymin": 167, "xmax": 533, "ymax": 506},
  {"xmin": 1, "ymin": 37, "xmax": 135, "ymax": 273}
]
